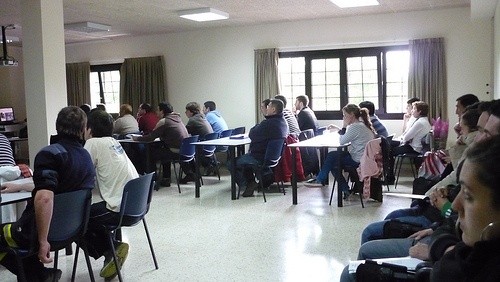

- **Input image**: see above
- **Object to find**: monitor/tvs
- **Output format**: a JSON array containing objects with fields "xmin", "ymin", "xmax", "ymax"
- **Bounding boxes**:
[{"xmin": 0, "ymin": 106, "xmax": 16, "ymax": 124}]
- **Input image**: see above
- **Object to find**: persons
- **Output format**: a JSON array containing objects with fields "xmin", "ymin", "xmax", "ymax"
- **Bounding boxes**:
[
  {"xmin": 0, "ymin": 105, "xmax": 96, "ymax": 282},
  {"xmin": 227, "ymin": 95, "xmax": 500, "ymax": 282},
  {"xmin": 75, "ymin": 110, "xmax": 140, "ymax": 280},
  {"xmin": 0, "ymin": 134, "xmax": 33, "ymax": 177},
  {"xmin": 80, "ymin": 101, "xmax": 230, "ymax": 187}
]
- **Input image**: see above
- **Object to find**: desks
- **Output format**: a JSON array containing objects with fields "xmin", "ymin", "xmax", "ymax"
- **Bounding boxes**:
[
  {"xmin": 285, "ymin": 134, "xmax": 351, "ymax": 208},
  {"xmin": 117, "ymin": 139, "xmax": 161, "ymax": 174},
  {"xmin": 189, "ymin": 133, "xmax": 251, "ymax": 201},
  {"xmin": 1, "ymin": 192, "xmax": 33, "ymax": 209}
]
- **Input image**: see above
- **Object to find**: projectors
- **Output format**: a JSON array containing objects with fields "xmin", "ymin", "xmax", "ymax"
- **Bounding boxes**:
[{"xmin": 0, "ymin": 57, "xmax": 19, "ymax": 66}]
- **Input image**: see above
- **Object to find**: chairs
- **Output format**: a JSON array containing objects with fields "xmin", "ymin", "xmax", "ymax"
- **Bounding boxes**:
[
  {"xmin": 70, "ymin": 171, "xmax": 159, "ymax": 282},
  {"xmin": 10, "ymin": 188, "xmax": 95, "ymax": 282},
  {"xmin": 120, "ymin": 127, "xmax": 431, "ymax": 209}
]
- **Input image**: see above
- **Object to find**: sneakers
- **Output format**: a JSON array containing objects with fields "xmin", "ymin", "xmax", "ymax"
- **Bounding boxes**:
[
  {"xmin": 344, "ymin": 190, "xmax": 353, "ymax": 200},
  {"xmin": 304, "ymin": 179, "xmax": 323, "ymax": 187}
]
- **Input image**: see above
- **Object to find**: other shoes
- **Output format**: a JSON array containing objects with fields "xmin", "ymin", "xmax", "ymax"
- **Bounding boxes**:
[
  {"xmin": 181, "ymin": 175, "xmax": 195, "ymax": 183},
  {"xmin": 243, "ymin": 182, "xmax": 257, "ymax": 197},
  {"xmin": 45, "ymin": 268, "xmax": 62, "ymax": 282},
  {"xmin": 100, "ymin": 241, "xmax": 129, "ymax": 282},
  {"xmin": 160, "ymin": 180, "xmax": 170, "ymax": 186},
  {"xmin": 367, "ymin": 198, "xmax": 381, "ymax": 203}
]
